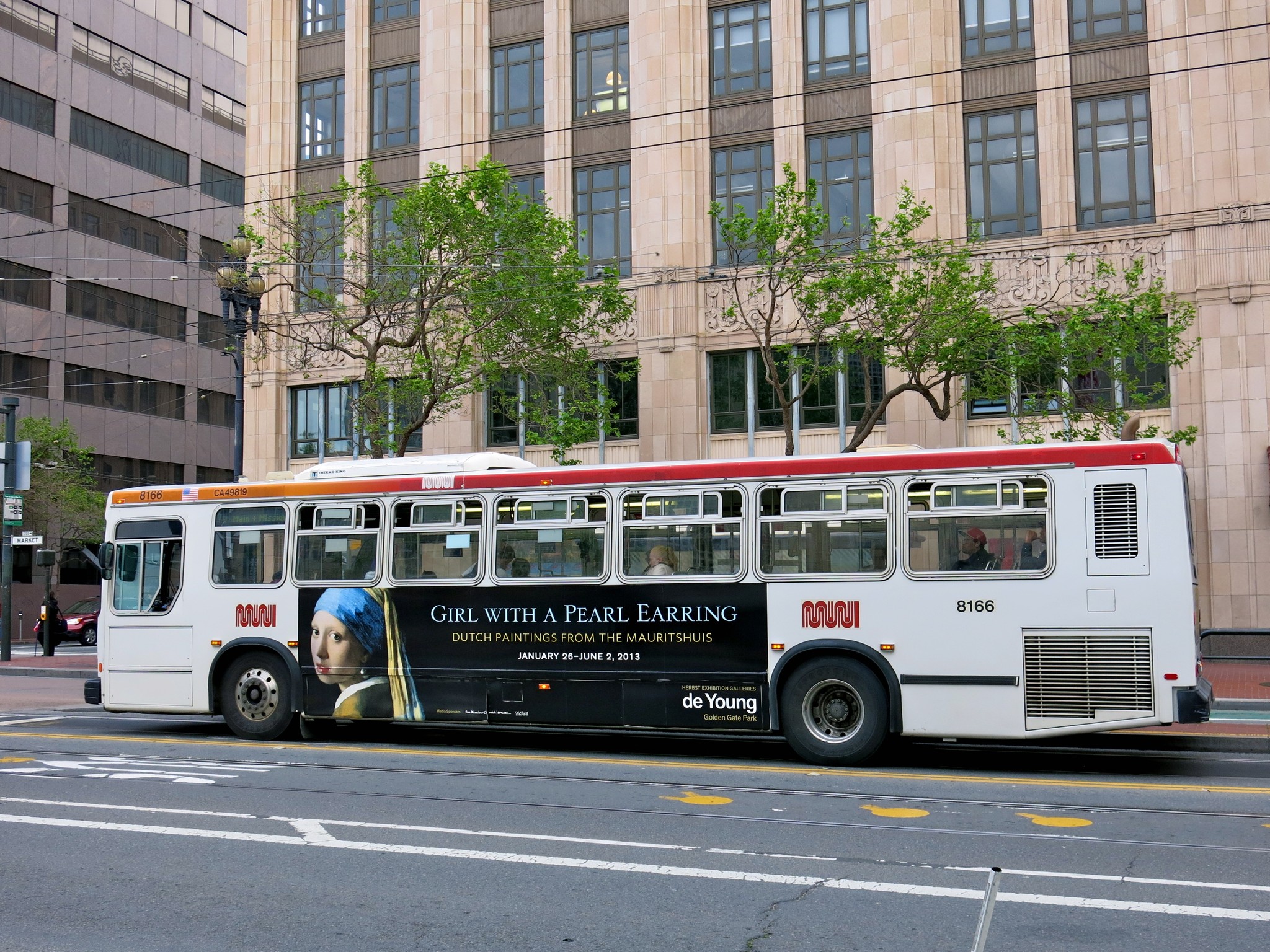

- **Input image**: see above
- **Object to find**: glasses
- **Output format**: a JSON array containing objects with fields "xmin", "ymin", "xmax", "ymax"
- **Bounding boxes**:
[{"xmin": 961, "ymin": 538, "xmax": 974, "ymax": 543}]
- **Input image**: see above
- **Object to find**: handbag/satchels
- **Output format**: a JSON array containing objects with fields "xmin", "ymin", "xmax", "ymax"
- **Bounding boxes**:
[{"xmin": 55, "ymin": 617, "xmax": 67, "ymax": 633}]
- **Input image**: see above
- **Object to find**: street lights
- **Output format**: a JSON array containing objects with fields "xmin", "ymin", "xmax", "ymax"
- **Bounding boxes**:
[{"xmin": 214, "ymin": 227, "xmax": 268, "ymax": 483}]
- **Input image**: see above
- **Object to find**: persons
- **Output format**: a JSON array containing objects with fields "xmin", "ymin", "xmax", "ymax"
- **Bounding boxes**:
[
  {"xmin": 643, "ymin": 545, "xmax": 678, "ymax": 575},
  {"xmin": 36, "ymin": 591, "xmax": 58, "ymax": 657},
  {"xmin": 951, "ymin": 528, "xmax": 994, "ymax": 570},
  {"xmin": 1021, "ymin": 520, "xmax": 1046, "ymax": 569},
  {"xmin": 459, "ymin": 542, "xmax": 530, "ymax": 578},
  {"xmin": 310, "ymin": 588, "xmax": 425, "ymax": 720}
]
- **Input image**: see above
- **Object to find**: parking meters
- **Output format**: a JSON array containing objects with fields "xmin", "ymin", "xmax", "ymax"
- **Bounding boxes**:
[{"xmin": 18, "ymin": 609, "xmax": 24, "ymax": 641}]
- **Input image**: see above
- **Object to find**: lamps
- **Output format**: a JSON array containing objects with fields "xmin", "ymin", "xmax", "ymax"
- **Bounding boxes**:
[
  {"xmin": 119, "ymin": 475, "xmax": 157, "ymax": 486},
  {"xmin": 606, "ymin": 65, "xmax": 627, "ymax": 85}
]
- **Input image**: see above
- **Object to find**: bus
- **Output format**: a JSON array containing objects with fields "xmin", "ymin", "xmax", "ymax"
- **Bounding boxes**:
[{"xmin": 87, "ymin": 416, "xmax": 1216, "ymax": 758}]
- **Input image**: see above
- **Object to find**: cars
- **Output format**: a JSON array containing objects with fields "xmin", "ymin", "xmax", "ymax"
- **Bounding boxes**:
[{"xmin": 56, "ymin": 596, "xmax": 100, "ymax": 647}]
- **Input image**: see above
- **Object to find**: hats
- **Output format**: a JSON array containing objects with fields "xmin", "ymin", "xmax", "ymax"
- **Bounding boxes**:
[
  {"xmin": 1038, "ymin": 520, "xmax": 1045, "ymax": 526},
  {"xmin": 959, "ymin": 528, "xmax": 987, "ymax": 546}
]
